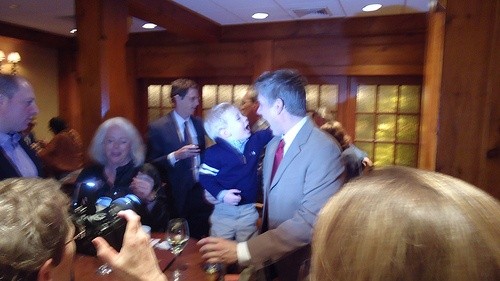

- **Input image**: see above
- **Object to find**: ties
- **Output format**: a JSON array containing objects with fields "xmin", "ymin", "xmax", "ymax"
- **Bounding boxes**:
[
  {"xmin": 184, "ymin": 121, "xmax": 194, "ymax": 169},
  {"xmin": 270, "ymin": 138, "xmax": 285, "ymax": 186}
]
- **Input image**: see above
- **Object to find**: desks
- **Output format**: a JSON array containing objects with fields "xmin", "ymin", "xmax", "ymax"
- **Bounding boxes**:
[{"xmin": 73, "ymin": 233, "xmax": 239, "ymax": 281}]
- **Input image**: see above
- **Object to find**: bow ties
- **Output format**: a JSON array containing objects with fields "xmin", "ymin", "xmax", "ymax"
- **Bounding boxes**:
[{"xmin": 11, "ymin": 134, "xmax": 22, "ymax": 148}]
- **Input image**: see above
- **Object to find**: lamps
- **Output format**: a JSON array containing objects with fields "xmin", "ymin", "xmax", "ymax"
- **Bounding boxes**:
[{"xmin": 0, "ymin": 49, "xmax": 21, "ymax": 76}]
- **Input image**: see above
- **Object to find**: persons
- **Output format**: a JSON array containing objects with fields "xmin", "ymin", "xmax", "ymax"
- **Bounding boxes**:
[{"xmin": 0, "ymin": 69, "xmax": 374, "ymax": 281}]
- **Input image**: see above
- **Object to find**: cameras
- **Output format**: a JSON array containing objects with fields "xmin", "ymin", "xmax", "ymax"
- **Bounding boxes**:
[{"xmin": 67, "ymin": 179, "xmax": 145, "ymax": 258}]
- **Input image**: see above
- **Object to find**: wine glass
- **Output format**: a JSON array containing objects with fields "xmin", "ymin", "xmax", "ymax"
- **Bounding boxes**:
[
  {"xmin": 190, "ymin": 143, "xmax": 199, "ymax": 170},
  {"xmin": 166, "ymin": 218, "xmax": 190, "ymax": 281}
]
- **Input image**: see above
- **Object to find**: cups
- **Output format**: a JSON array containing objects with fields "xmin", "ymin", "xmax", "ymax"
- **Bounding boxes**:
[{"xmin": 203, "ymin": 249, "xmax": 220, "ymax": 273}]
